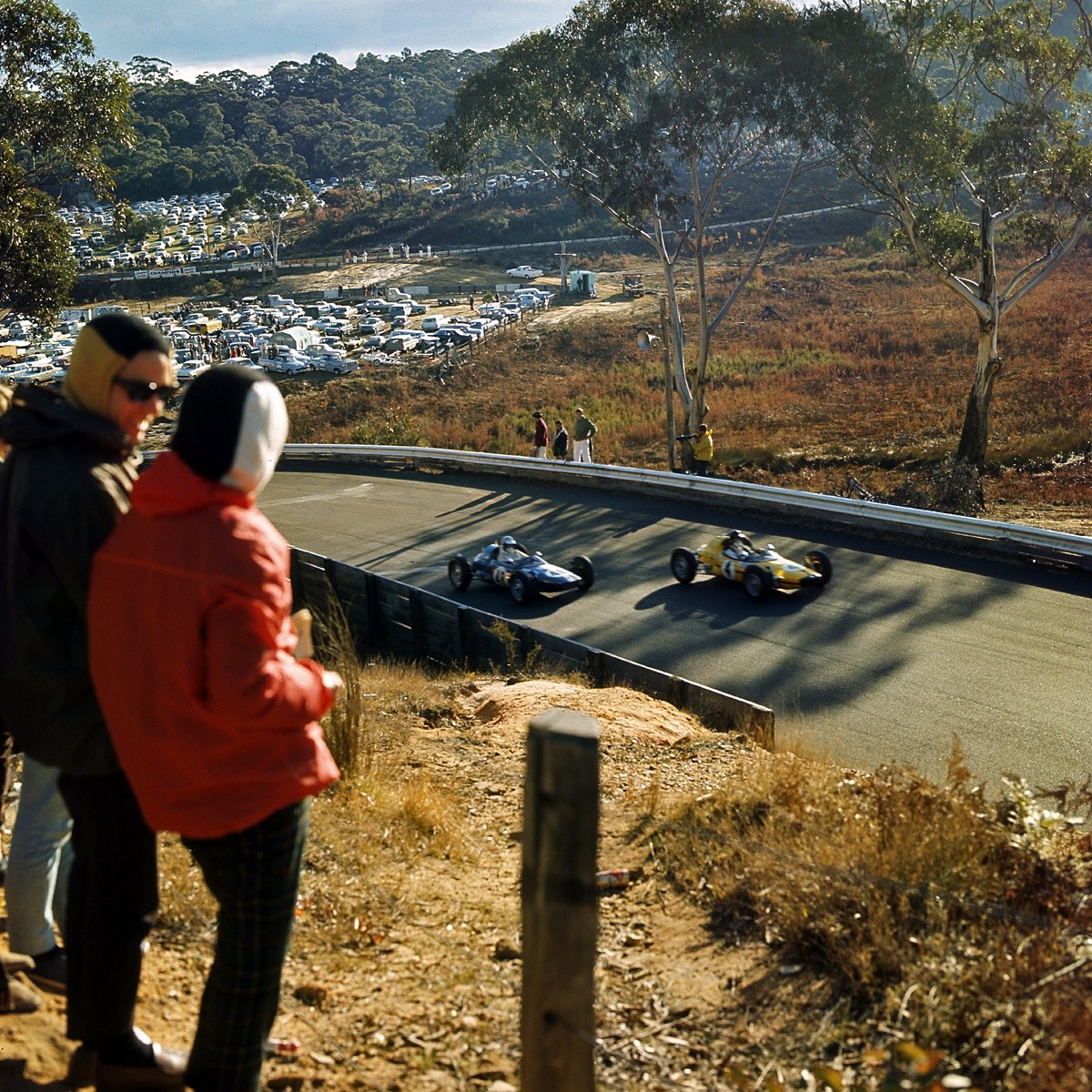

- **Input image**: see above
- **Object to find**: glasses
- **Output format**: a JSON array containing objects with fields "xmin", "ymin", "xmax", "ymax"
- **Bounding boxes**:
[{"xmin": 113, "ymin": 376, "xmax": 176, "ymax": 403}]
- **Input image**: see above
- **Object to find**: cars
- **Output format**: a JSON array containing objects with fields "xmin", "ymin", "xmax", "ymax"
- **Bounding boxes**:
[
  {"xmin": 69, "ymin": 288, "xmax": 547, "ymax": 381},
  {"xmin": 54, "ymin": 161, "xmax": 571, "ymax": 268}
]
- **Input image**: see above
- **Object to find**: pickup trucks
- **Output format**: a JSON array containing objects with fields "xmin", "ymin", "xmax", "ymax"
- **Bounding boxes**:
[{"xmin": 506, "ymin": 265, "xmax": 544, "ymax": 280}]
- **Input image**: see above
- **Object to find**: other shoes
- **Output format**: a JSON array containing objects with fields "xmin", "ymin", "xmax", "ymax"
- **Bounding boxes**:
[
  {"xmin": 26, "ymin": 946, "xmax": 70, "ymax": 990},
  {"xmin": 83, "ymin": 1027, "xmax": 187, "ymax": 1086}
]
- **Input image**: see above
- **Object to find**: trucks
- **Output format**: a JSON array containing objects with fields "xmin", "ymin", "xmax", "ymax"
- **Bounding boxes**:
[{"xmin": 622, "ymin": 272, "xmax": 645, "ymax": 297}]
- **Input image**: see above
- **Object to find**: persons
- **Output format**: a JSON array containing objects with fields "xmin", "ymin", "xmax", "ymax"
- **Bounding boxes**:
[
  {"xmin": 550, "ymin": 419, "xmax": 570, "ymax": 461},
  {"xmin": 85, "ymin": 362, "xmax": 349, "ymax": 1092},
  {"xmin": 572, "ymin": 407, "xmax": 598, "ymax": 464},
  {"xmin": 1, "ymin": 311, "xmax": 194, "ymax": 1091},
  {"xmin": 722, "ymin": 533, "xmax": 762, "ymax": 562},
  {"xmin": 687, "ymin": 423, "xmax": 714, "ymax": 477},
  {"xmin": 532, "ymin": 411, "xmax": 549, "ymax": 460},
  {"xmin": 0, "ymin": 378, "xmax": 80, "ymax": 993},
  {"xmin": 1, "ymin": 240, "xmax": 584, "ymax": 380},
  {"xmin": 497, "ymin": 535, "xmax": 531, "ymax": 562}
]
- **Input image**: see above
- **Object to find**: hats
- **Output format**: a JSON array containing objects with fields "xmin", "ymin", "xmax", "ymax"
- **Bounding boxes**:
[
  {"xmin": 161, "ymin": 361, "xmax": 290, "ymax": 504},
  {"xmin": 66, "ymin": 310, "xmax": 173, "ymax": 421}
]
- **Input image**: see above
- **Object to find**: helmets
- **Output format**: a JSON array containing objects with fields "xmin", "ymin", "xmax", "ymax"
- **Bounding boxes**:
[
  {"xmin": 698, "ymin": 424, "xmax": 709, "ymax": 431},
  {"xmin": 730, "ymin": 529, "xmax": 742, "ymax": 538},
  {"xmin": 500, "ymin": 536, "xmax": 516, "ymax": 545}
]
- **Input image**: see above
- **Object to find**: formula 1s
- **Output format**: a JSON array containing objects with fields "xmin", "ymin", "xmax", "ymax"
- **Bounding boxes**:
[
  {"xmin": 670, "ymin": 530, "xmax": 832, "ymax": 603},
  {"xmin": 448, "ymin": 539, "xmax": 595, "ymax": 605}
]
